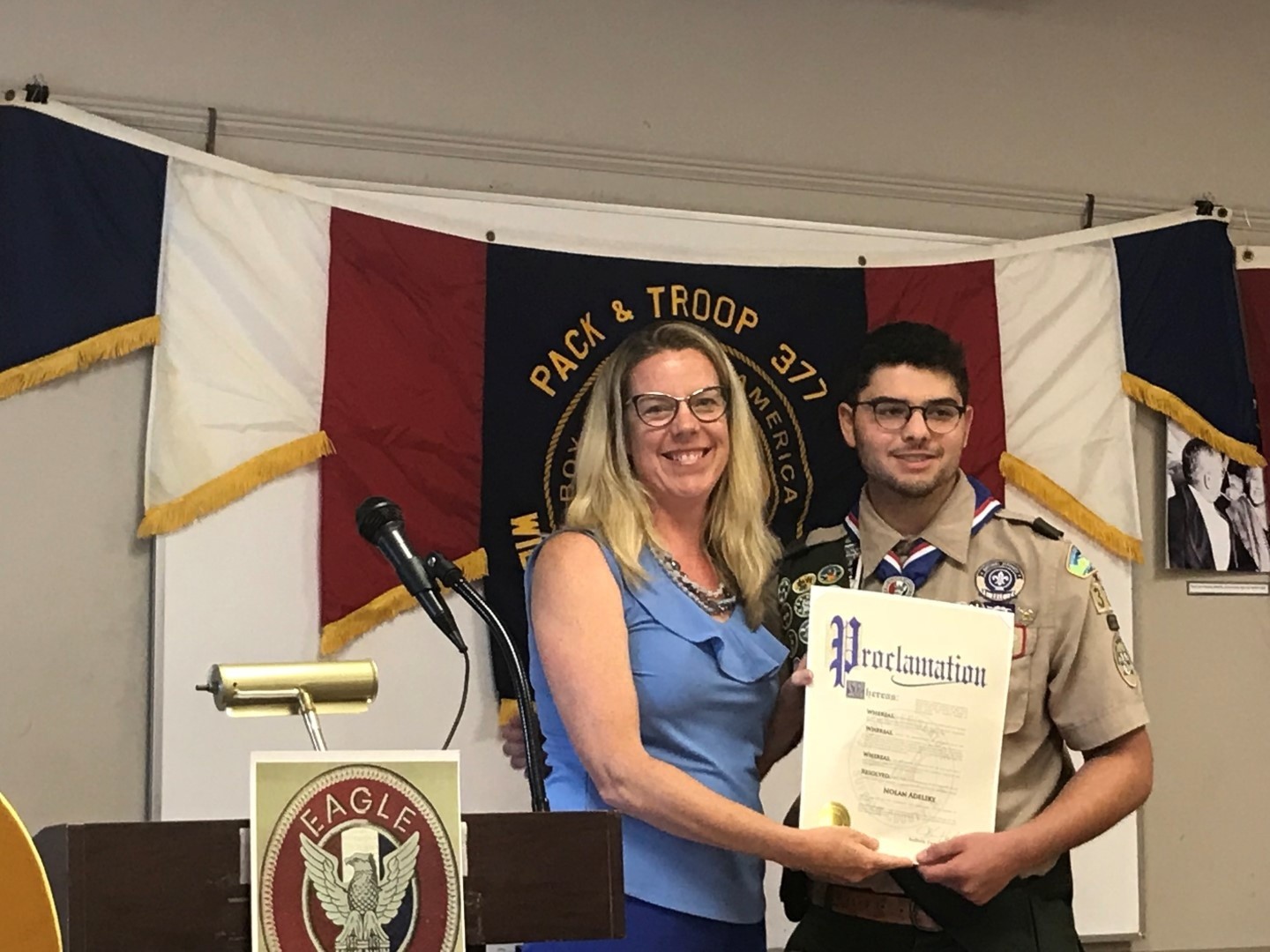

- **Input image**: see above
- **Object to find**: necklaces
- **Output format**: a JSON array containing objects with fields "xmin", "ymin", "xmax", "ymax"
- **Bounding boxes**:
[{"xmin": 641, "ymin": 537, "xmax": 737, "ymax": 616}]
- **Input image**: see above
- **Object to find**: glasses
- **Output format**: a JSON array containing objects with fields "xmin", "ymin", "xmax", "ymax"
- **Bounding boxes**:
[
  {"xmin": 851, "ymin": 397, "xmax": 967, "ymax": 433},
  {"xmin": 624, "ymin": 384, "xmax": 730, "ymax": 427}
]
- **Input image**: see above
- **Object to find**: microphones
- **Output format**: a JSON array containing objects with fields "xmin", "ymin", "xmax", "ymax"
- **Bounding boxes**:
[{"xmin": 357, "ymin": 496, "xmax": 468, "ymax": 652}]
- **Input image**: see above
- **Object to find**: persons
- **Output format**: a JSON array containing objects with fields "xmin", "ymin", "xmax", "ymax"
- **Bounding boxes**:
[
  {"xmin": 523, "ymin": 318, "xmax": 914, "ymax": 952},
  {"xmin": 779, "ymin": 321, "xmax": 1154, "ymax": 952},
  {"xmin": 1164, "ymin": 437, "xmax": 1270, "ymax": 573}
]
